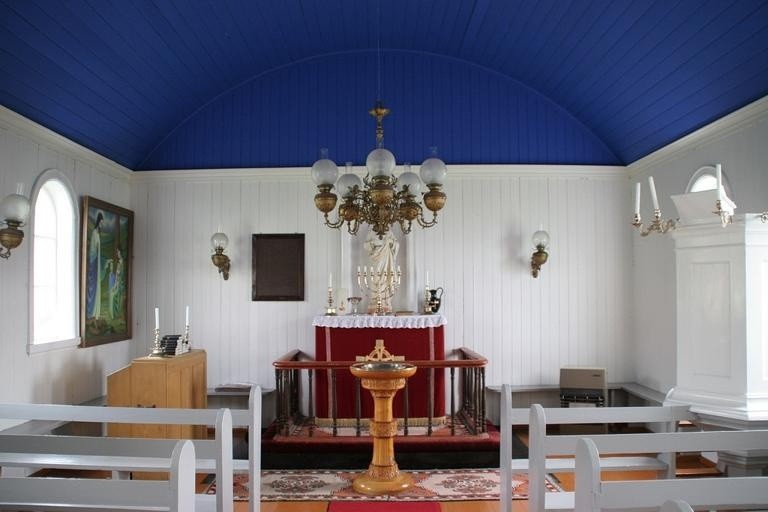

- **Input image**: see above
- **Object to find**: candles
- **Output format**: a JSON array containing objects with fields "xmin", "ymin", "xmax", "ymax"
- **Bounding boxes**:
[
  {"xmin": 634, "ymin": 182, "xmax": 641, "ymax": 214},
  {"xmin": 185, "ymin": 305, "xmax": 190, "ymax": 323},
  {"xmin": 356, "ymin": 264, "xmax": 401, "ymax": 285},
  {"xmin": 714, "ymin": 162, "xmax": 721, "ymax": 200},
  {"xmin": 329, "ymin": 270, "xmax": 332, "ymax": 287},
  {"xmin": 425, "ymin": 272, "xmax": 430, "ymax": 287},
  {"xmin": 647, "ymin": 175, "xmax": 660, "ymax": 212},
  {"xmin": 154, "ymin": 307, "xmax": 160, "ymax": 328}
]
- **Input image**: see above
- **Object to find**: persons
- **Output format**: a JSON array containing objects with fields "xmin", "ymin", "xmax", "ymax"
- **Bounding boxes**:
[
  {"xmin": 108, "ymin": 262, "xmax": 116, "ymax": 291},
  {"xmin": 109, "ymin": 246, "xmax": 125, "ymax": 320},
  {"xmin": 86, "ymin": 212, "xmax": 114, "ymax": 320},
  {"xmin": 363, "ymin": 225, "xmax": 399, "ymax": 312}
]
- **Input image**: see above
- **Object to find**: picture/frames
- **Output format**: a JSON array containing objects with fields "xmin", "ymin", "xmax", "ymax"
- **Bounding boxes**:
[
  {"xmin": 78, "ymin": 194, "xmax": 133, "ymax": 349},
  {"xmin": 251, "ymin": 233, "xmax": 306, "ymax": 303}
]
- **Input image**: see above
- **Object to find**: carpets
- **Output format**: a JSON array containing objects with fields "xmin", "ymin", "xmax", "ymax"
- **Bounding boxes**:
[{"xmin": 202, "ymin": 460, "xmax": 570, "ymax": 498}]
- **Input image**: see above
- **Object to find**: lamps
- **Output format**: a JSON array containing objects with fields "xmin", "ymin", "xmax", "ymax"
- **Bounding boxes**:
[
  {"xmin": 309, "ymin": 0, "xmax": 449, "ymax": 243},
  {"xmin": 529, "ymin": 230, "xmax": 550, "ymax": 280},
  {"xmin": 0, "ymin": 194, "xmax": 31, "ymax": 260},
  {"xmin": 211, "ymin": 232, "xmax": 231, "ymax": 281}
]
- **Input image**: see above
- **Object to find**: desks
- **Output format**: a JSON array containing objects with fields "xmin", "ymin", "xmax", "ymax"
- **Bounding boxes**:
[
  {"xmin": 313, "ymin": 313, "xmax": 446, "ymax": 426},
  {"xmin": 105, "ymin": 350, "xmax": 208, "ymax": 484}
]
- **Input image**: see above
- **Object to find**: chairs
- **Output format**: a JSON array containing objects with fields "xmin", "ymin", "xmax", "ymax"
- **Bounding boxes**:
[{"xmin": 558, "ymin": 367, "xmax": 624, "ymax": 434}]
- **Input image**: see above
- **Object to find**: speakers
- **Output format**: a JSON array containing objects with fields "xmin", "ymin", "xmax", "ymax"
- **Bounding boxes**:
[{"xmin": 559, "ymin": 368, "xmax": 608, "ymax": 436}]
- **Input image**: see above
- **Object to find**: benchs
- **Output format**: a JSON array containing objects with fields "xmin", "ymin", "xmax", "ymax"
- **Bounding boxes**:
[
  {"xmin": 528, "ymin": 402, "xmax": 767, "ymax": 511},
  {"xmin": 0, "ymin": 382, "xmax": 264, "ymax": 511},
  {"xmin": 0, "ymin": 439, "xmax": 197, "ymax": 512},
  {"xmin": 572, "ymin": 437, "xmax": 767, "ymax": 511},
  {"xmin": 496, "ymin": 382, "xmax": 677, "ymax": 512},
  {"xmin": 0, "ymin": 406, "xmax": 236, "ymax": 512}
]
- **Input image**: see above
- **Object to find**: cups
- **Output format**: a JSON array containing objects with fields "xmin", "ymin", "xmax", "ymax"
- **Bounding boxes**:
[{"xmin": 336, "ymin": 287, "xmax": 349, "ymax": 315}]
